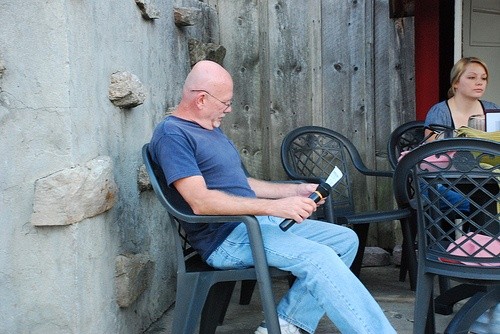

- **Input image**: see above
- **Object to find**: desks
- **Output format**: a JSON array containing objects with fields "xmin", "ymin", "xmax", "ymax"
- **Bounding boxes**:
[{"xmin": 419, "ymin": 170, "xmax": 500, "ymax": 293}]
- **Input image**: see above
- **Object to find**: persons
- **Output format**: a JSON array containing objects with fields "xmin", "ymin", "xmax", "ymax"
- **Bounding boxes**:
[
  {"xmin": 147, "ymin": 60, "xmax": 399, "ymax": 334},
  {"xmin": 425, "ymin": 57, "xmax": 500, "ymax": 212}
]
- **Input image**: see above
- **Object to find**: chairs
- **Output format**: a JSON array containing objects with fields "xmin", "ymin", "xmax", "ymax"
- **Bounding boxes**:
[
  {"xmin": 388, "ymin": 120, "xmax": 500, "ymax": 334},
  {"xmin": 142, "ymin": 142, "xmax": 322, "ymax": 334},
  {"xmin": 280, "ymin": 126, "xmax": 417, "ymax": 289}
]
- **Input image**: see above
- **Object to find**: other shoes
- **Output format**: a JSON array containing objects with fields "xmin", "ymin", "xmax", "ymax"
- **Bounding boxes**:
[{"xmin": 255, "ymin": 322, "xmax": 306, "ymax": 334}]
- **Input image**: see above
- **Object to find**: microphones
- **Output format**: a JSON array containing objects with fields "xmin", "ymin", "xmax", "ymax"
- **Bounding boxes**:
[{"xmin": 279, "ymin": 183, "xmax": 332, "ymax": 232}]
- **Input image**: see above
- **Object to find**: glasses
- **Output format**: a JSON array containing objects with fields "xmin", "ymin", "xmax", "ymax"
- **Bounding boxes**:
[{"xmin": 191, "ymin": 90, "xmax": 232, "ymax": 111}]
